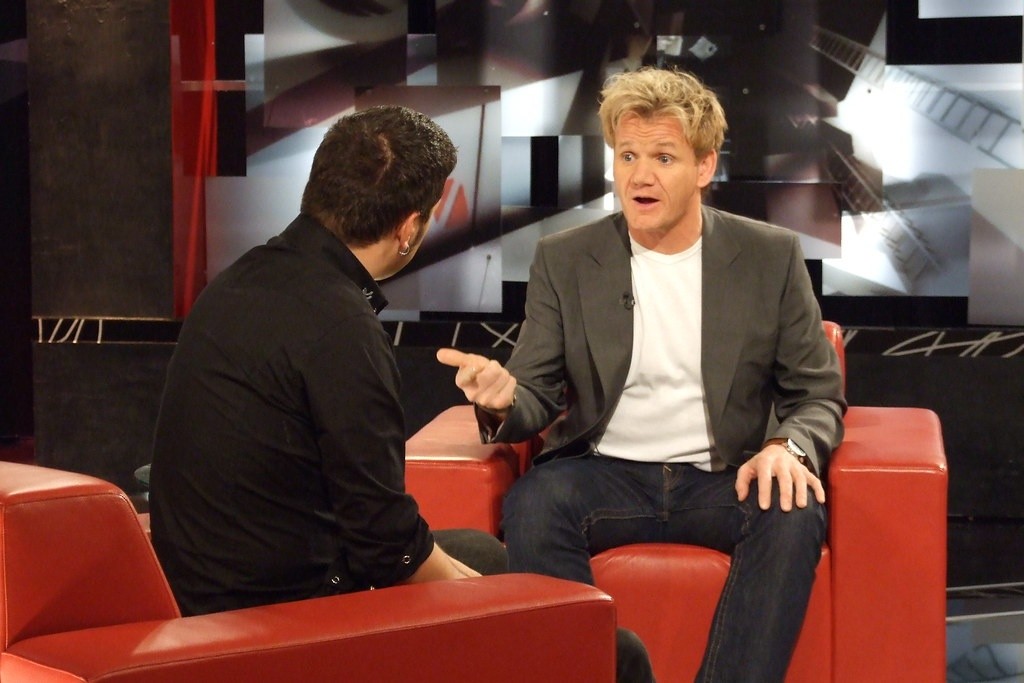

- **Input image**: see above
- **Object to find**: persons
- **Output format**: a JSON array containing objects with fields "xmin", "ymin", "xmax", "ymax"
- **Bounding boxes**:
[
  {"xmin": 437, "ymin": 68, "xmax": 847, "ymax": 683},
  {"xmin": 148, "ymin": 106, "xmax": 656, "ymax": 682}
]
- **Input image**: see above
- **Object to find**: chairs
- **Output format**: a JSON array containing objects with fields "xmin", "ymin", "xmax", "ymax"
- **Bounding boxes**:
[{"xmin": 0, "ymin": 319, "xmax": 948, "ymax": 683}]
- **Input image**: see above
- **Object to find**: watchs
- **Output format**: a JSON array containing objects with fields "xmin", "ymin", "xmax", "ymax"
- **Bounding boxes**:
[{"xmin": 761, "ymin": 438, "xmax": 807, "ymax": 463}]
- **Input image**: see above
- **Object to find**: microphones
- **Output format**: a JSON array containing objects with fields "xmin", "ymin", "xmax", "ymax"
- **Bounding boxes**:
[{"xmin": 622, "ymin": 292, "xmax": 637, "ymax": 310}]
